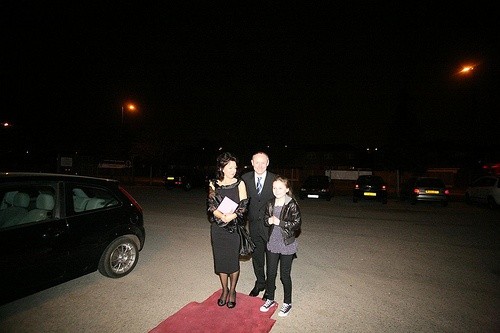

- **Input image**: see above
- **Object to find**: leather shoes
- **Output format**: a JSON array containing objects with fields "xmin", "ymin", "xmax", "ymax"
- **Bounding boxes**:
[
  {"xmin": 249, "ymin": 286, "xmax": 260, "ymax": 297},
  {"xmin": 262, "ymin": 290, "xmax": 269, "ymax": 301}
]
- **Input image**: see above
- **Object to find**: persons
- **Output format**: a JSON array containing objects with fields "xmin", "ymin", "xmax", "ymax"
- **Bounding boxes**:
[
  {"xmin": 241, "ymin": 152, "xmax": 302, "ymax": 316},
  {"xmin": 207, "ymin": 151, "xmax": 249, "ymax": 308}
]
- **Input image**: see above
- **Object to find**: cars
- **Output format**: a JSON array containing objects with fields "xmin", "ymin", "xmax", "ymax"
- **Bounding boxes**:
[
  {"xmin": 400, "ymin": 175, "xmax": 449, "ymax": 207},
  {"xmin": 164, "ymin": 169, "xmax": 210, "ymax": 192},
  {"xmin": 300, "ymin": 176, "xmax": 335, "ymax": 201},
  {"xmin": 464, "ymin": 176, "xmax": 500, "ymax": 210},
  {"xmin": 353, "ymin": 175, "xmax": 387, "ymax": 205}
]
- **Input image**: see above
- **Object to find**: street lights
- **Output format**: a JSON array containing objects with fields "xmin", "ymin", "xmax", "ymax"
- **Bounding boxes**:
[
  {"xmin": 365, "ymin": 146, "xmax": 378, "ymax": 171},
  {"xmin": 121, "ymin": 103, "xmax": 136, "ymax": 133}
]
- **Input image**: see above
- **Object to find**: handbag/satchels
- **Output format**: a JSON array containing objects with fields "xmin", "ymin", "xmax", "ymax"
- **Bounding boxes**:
[{"xmin": 239, "ymin": 225, "xmax": 256, "ymax": 262}]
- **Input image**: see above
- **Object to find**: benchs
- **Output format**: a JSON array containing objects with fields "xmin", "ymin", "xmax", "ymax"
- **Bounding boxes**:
[{"xmin": 73, "ymin": 196, "xmax": 112, "ymax": 212}]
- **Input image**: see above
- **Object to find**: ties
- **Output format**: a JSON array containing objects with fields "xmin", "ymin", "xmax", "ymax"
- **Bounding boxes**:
[{"xmin": 256, "ymin": 177, "xmax": 262, "ymax": 197}]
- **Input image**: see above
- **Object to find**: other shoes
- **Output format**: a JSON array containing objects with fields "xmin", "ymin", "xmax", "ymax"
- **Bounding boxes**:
[
  {"xmin": 227, "ymin": 291, "xmax": 236, "ymax": 308},
  {"xmin": 218, "ymin": 289, "xmax": 229, "ymax": 306}
]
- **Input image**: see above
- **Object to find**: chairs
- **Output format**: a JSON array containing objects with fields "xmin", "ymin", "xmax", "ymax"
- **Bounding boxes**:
[{"xmin": 0, "ymin": 191, "xmax": 54, "ymax": 228}]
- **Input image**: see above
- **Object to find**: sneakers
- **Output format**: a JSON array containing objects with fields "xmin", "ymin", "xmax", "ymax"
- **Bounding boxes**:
[
  {"xmin": 260, "ymin": 298, "xmax": 275, "ymax": 312},
  {"xmin": 278, "ymin": 303, "xmax": 293, "ymax": 317}
]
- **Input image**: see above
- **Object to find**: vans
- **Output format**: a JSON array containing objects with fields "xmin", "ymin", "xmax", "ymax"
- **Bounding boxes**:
[{"xmin": 0, "ymin": 172, "xmax": 146, "ymax": 305}]
